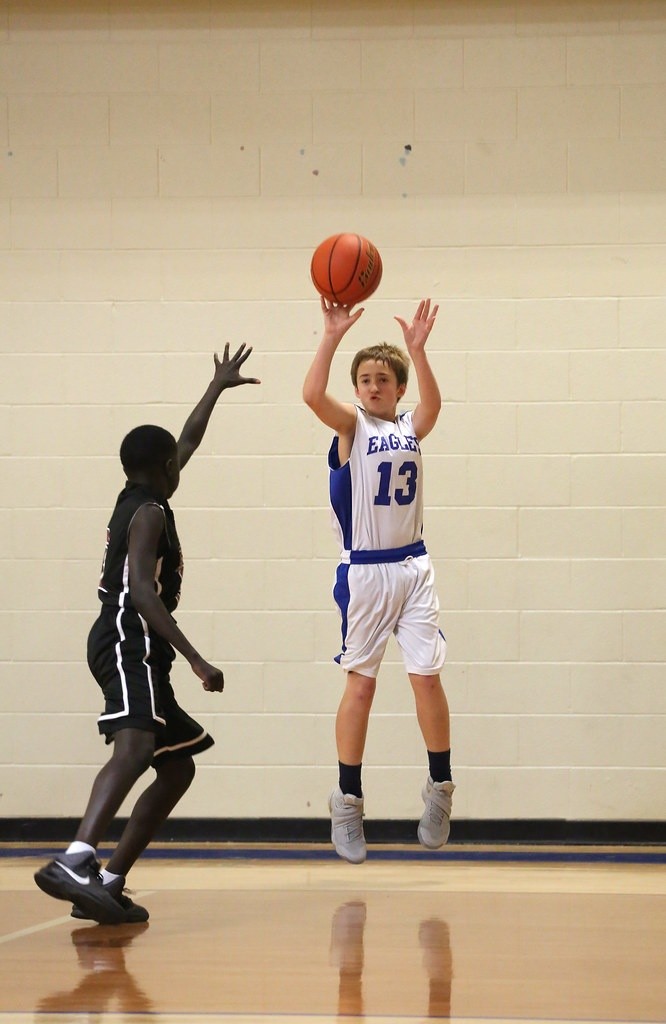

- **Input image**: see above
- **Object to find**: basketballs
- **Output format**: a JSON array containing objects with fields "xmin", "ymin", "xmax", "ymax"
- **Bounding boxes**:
[{"xmin": 312, "ymin": 232, "xmax": 382, "ymax": 305}]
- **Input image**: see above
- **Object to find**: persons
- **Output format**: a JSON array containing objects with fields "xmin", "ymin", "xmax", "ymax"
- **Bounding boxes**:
[
  {"xmin": 302, "ymin": 292, "xmax": 456, "ymax": 865},
  {"xmin": 32, "ymin": 342, "xmax": 263, "ymax": 927},
  {"xmin": 328, "ymin": 901, "xmax": 453, "ymax": 1024},
  {"xmin": 32, "ymin": 922, "xmax": 152, "ymax": 1014}
]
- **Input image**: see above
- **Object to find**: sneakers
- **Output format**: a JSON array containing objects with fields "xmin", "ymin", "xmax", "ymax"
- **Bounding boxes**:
[
  {"xmin": 70, "ymin": 923, "xmax": 150, "ymax": 968},
  {"xmin": 417, "ymin": 776, "xmax": 456, "ymax": 849},
  {"xmin": 329, "ymin": 900, "xmax": 366, "ymax": 968},
  {"xmin": 34, "ymin": 850, "xmax": 127, "ymax": 925},
  {"xmin": 419, "ymin": 918, "xmax": 452, "ymax": 981},
  {"xmin": 327, "ymin": 784, "xmax": 367, "ymax": 864},
  {"xmin": 71, "ymin": 876, "xmax": 150, "ymax": 926}
]
